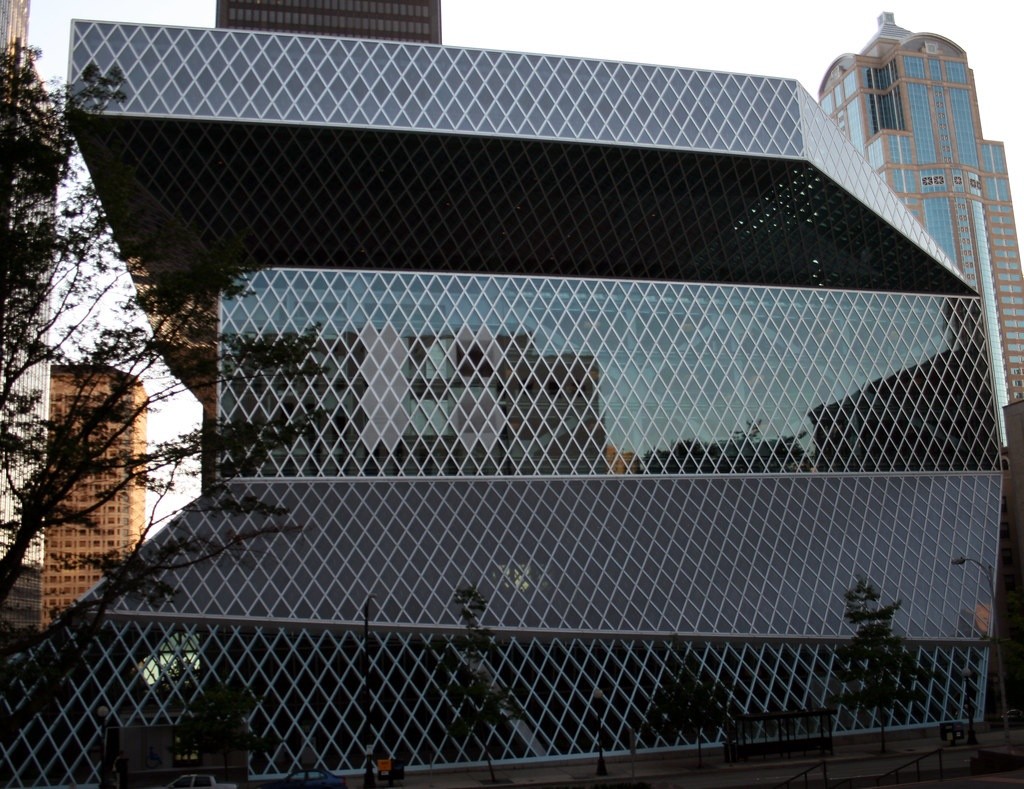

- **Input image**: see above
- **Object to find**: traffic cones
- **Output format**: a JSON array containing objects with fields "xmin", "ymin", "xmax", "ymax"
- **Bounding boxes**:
[{"xmin": 950, "ymin": 555, "xmax": 1014, "ymax": 756}]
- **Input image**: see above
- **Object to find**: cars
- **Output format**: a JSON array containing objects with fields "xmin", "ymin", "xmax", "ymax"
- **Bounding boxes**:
[
  {"xmin": 255, "ymin": 769, "xmax": 348, "ymax": 789},
  {"xmin": 145, "ymin": 773, "xmax": 239, "ymax": 789}
]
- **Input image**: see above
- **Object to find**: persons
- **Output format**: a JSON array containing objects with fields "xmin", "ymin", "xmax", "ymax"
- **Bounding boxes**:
[{"xmin": 112, "ymin": 751, "xmax": 129, "ymax": 789}]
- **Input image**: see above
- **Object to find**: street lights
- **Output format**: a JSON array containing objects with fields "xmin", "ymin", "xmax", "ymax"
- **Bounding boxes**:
[
  {"xmin": 592, "ymin": 688, "xmax": 607, "ymax": 775},
  {"xmin": 95, "ymin": 704, "xmax": 110, "ymax": 786},
  {"xmin": 362, "ymin": 589, "xmax": 378, "ymax": 789}
]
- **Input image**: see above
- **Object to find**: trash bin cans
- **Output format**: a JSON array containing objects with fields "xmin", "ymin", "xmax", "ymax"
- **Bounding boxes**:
[
  {"xmin": 940, "ymin": 721, "xmax": 966, "ymax": 746},
  {"xmin": 376, "ymin": 757, "xmax": 406, "ymax": 787}
]
